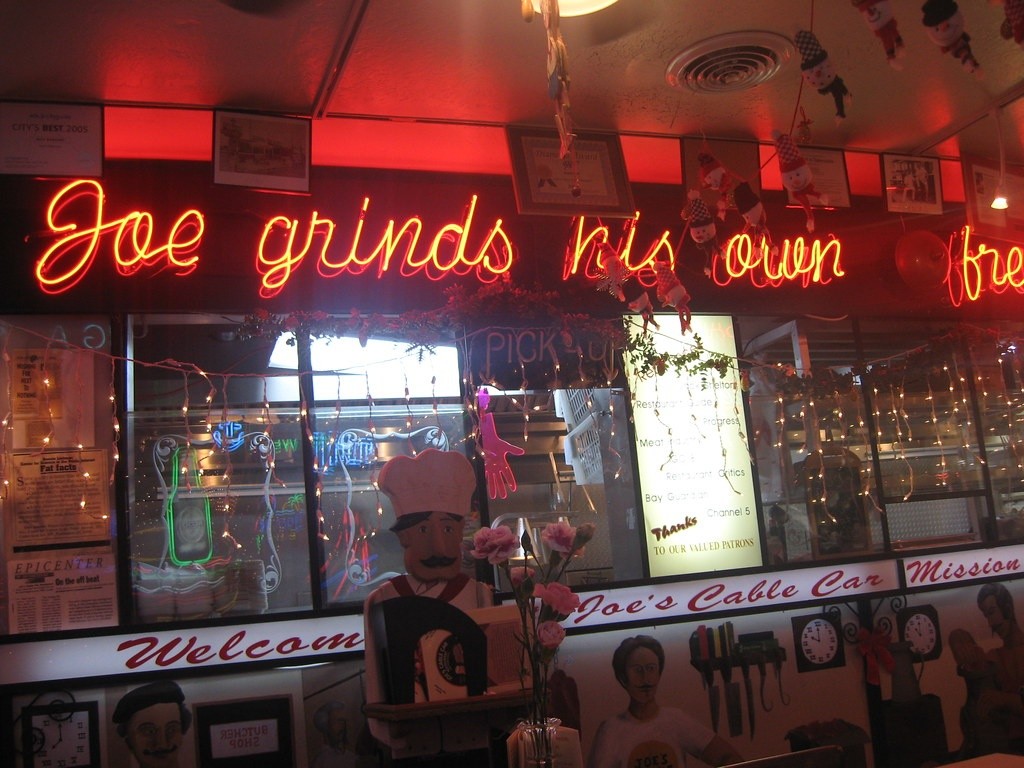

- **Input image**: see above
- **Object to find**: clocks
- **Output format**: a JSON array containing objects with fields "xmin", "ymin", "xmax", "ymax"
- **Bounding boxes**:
[
  {"xmin": 22, "ymin": 700, "xmax": 101, "ymax": 768},
  {"xmin": 896, "ymin": 605, "xmax": 943, "ymax": 664},
  {"xmin": 791, "ymin": 611, "xmax": 846, "ymax": 673}
]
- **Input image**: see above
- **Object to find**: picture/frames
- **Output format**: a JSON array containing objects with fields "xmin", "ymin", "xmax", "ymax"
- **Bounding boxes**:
[
  {"xmin": 504, "ymin": 123, "xmax": 635, "ymax": 218},
  {"xmin": 1, "ymin": 99, "xmax": 104, "ymax": 179},
  {"xmin": 783, "ymin": 146, "xmax": 852, "ymax": 210},
  {"xmin": 961, "ymin": 153, "xmax": 1024, "ymax": 245},
  {"xmin": 212, "ymin": 107, "xmax": 312, "ymax": 196},
  {"xmin": 192, "ymin": 694, "xmax": 296, "ymax": 767},
  {"xmin": 679, "ymin": 138, "xmax": 762, "ymax": 214},
  {"xmin": 879, "ymin": 153, "xmax": 945, "ymax": 216}
]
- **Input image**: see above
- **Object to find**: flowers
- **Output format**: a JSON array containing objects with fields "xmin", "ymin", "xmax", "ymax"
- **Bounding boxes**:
[{"xmin": 471, "ymin": 524, "xmax": 598, "ymax": 767}]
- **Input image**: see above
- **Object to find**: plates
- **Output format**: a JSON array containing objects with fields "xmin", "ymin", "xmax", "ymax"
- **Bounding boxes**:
[{"xmin": 131, "ymin": 559, "xmax": 269, "ymax": 617}]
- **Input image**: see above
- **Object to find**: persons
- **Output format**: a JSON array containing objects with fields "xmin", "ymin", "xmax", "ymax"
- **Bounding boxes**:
[
  {"xmin": 364, "ymin": 449, "xmax": 493, "ymax": 768},
  {"xmin": 902, "ymin": 162, "xmax": 929, "ymax": 202}
]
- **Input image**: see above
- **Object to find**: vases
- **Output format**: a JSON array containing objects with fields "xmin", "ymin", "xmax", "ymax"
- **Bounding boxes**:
[{"xmin": 515, "ymin": 719, "xmax": 561, "ymax": 767}]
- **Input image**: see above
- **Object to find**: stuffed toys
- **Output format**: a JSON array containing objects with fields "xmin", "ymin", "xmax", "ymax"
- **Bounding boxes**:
[{"xmin": 681, "ymin": 127, "xmax": 829, "ymax": 275}]
